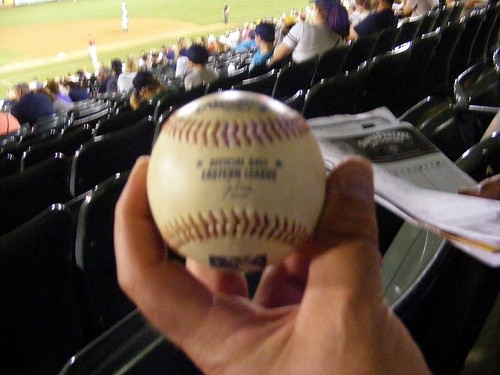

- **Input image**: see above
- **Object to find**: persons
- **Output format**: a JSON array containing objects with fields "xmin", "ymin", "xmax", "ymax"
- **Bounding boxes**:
[
  {"xmin": 113, "ymin": 89, "xmax": 500, "ymax": 375},
  {"xmin": 0, "ymin": 0, "xmax": 490, "ymax": 139}
]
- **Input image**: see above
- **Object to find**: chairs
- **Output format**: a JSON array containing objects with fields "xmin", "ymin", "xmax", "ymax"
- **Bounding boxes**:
[{"xmin": 0, "ymin": 0, "xmax": 500, "ymax": 375}]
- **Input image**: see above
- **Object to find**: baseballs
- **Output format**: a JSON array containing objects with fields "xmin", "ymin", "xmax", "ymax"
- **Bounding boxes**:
[{"xmin": 146, "ymin": 90, "xmax": 326, "ymax": 272}]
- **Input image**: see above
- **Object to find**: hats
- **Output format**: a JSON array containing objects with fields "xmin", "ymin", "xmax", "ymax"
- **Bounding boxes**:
[
  {"xmin": 187, "ymin": 46, "xmax": 208, "ymax": 64},
  {"xmin": 256, "ymin": 24, "xmax": 275, "ymax": 39},
  {"xmin": 133, "ymin": 73, "xmax": 149, "ymax": 96}
]
